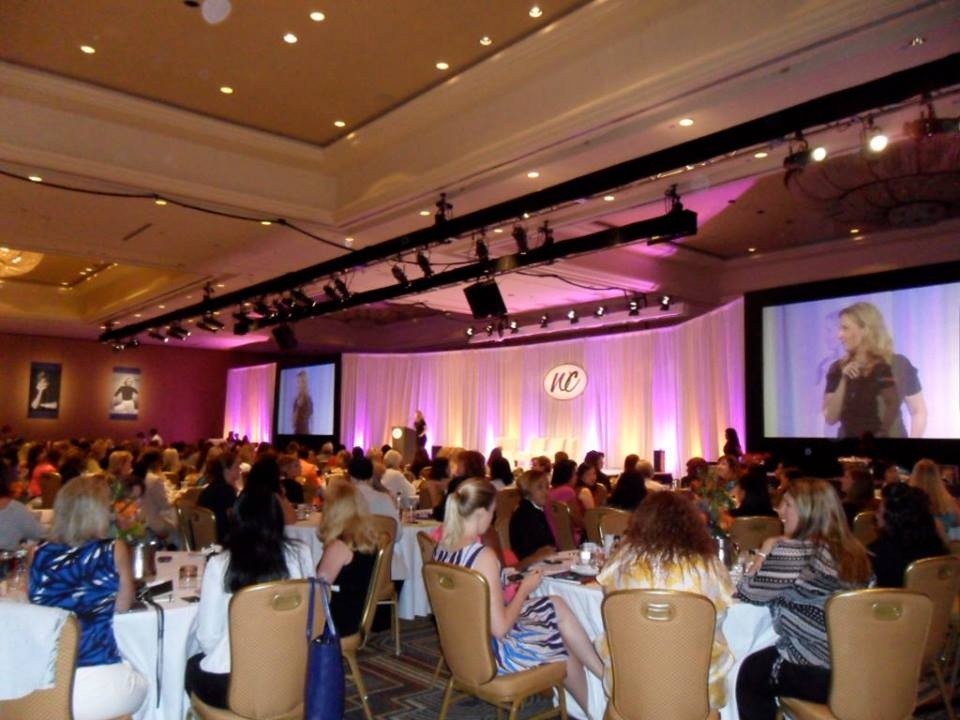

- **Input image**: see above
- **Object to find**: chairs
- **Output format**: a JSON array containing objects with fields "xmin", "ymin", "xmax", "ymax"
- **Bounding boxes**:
[{"xmin": 1, "ymin": 453, "xmax": 960, "ymax": 720}]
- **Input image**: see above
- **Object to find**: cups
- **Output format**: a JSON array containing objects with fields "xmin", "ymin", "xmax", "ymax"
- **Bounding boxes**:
[
  {"xmin": 579, "ymin": 542, "xmax": 596, "ymax": 566},
  {"xmin": 178, "ymin": 564, "xmax": 199, "ymax": 589}
]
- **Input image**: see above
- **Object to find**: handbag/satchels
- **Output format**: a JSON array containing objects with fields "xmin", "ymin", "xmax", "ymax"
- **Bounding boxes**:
[{"xmin": 305, "ymin": 577, "xmax": 345, "ymax": 720}]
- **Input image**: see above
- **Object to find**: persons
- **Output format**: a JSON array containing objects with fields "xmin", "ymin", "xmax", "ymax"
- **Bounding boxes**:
[
  {"xmin": 1, "ymin": 427, "xmax": 960, "ymax": 720},
  {"xmin": 288, "ymin": 371, "xmax": 315, "ymax": 434},
  {"xmin": 822, "ymin": 302, "xmax": 928, "ymax": 439},
  {"xmin": 30, "ymin": 369, "xmax": 58, "ymax": 411},
  {"xmin": 412, "ymin": 410, "xmax": 427, "ymax": 450},
  {"xmin": 114, "ymin": 376, "xmax": 139, "ymax": 411}
]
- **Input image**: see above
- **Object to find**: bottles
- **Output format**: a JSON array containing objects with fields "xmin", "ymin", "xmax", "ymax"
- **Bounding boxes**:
[{"xmin": 393, "ymin": 491, "xmax": 402, "ymax": 525}]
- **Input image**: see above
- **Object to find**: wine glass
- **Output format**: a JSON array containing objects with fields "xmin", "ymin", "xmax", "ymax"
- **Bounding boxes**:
[{"xmin": 402, "ymin": 495, "xmax": 420, "ymax": 524}]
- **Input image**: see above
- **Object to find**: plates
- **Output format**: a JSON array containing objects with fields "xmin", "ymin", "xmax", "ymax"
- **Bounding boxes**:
[{"xmin": 570, "ymin": 565, "xmax": 601, "ymax": 576}]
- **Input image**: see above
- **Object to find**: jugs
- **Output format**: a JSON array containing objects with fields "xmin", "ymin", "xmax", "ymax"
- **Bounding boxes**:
[
  {"xmin": 130, "ymin": 536, "xmax": 159, "ymax": 586},
  {"xmin": 710, "ymin": 535, "xmax": 740, "ymax": 572}
]
